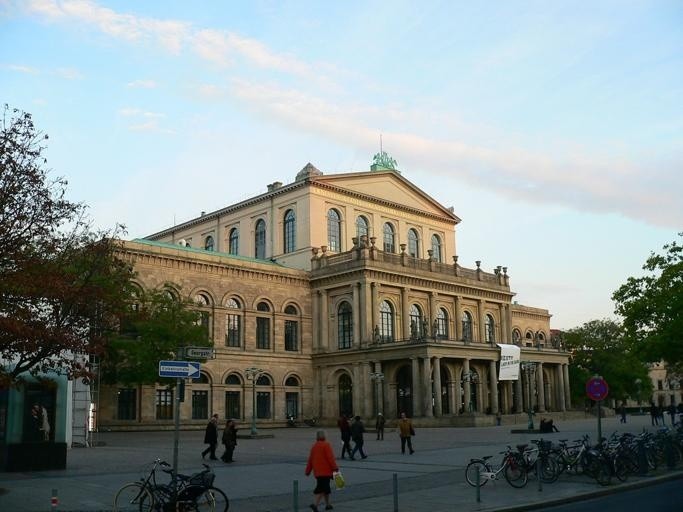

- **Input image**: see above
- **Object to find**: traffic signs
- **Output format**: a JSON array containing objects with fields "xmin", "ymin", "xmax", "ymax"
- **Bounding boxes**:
[
  {"xmin": 158, "ymin": 360, "xmax": 200, "ymax": 379},
  {"xmin": 184, "ymin": 346, "xmax": 217, "ymax": 360}
]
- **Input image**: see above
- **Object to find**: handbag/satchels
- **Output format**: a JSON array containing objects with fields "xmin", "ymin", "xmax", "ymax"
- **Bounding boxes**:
[
  {"xmin": 335, "ymin": 473, "xmax": 344, "ymax": 489},
  {"xmin": 410, "ymin": 429, "xmax": 416, "ymax": 436}
]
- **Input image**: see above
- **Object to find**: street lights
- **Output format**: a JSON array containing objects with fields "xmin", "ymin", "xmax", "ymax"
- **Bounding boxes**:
[
  {"xmin": 244, "ymin": 366, "xmax": 263, "ymax": 434},
  {"xmin": 519, "ymin": 358, "xmax": 536, "ymax": 429},
  {"xmin": 367, "ymin": 370, "xmax": 385, "ymax": 414},
  {"xmin": 634, "ymin": 378, "xmax": 643, "ymax": 413}
]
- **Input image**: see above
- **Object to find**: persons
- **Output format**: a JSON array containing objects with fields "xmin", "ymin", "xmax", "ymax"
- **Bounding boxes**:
[
  {"xmin": 433, "ymin": 319, "xmax": 438, "ymax": 343},
  {"xmin": 375, "ymin": 412, "xmax": 385, "ymax": 441},
  {"xmin": 337, "ymin": 411, "xmax": 352, "ymax": 459},
  {"xmin": 374, "ymin": 325, "xmax": 379, "ymax": 336},
  {"xmin": 540, "ymin": 418, "xmax": 547, "ymax": 431},
  {"xmin": 423, "ymin": 318, "xmax": 429, "ymax": 337},
  {"xmin": 31, "ymin": 409, "xmax": 40, "ymax": 431},
  {"xmin": 34, "ymin": 405, "xmax": 40, "ymax": 417},
  {"xmin": 398, "ymin": 412, "xmax": 415, "ymax": 455},
  {"xmin": 410, "ymin": 320, "xmax": 417, "ymax": 338},
  {"xmin": 202, "ymin": 413, "xmax": 238, "ymax": 464},
  {"xmin": 304, "ymin": 431, "xmax": 340, "ymax": 512},
  {"xmin": 547, "ymin": 420, "xmax": 560, "ymax": 432},
  {"xmin": 349, "ymin": 416, "xmax": 367, "ymax": 461},
  {"xmin": 495, "ymin": 409, "xmax": 502, "ymax": 426},
  {"xmin": 619, "ymin": 403, "xmax": 628, "ymax": 424},
  {"xmin": 649, "ymin": 401, "xmax": 683, "ymax": 427}
]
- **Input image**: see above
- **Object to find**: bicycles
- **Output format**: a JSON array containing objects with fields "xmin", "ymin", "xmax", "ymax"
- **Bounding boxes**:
[
  {"xmin": 464, "ymin": 447, "xmax": 529, "ymax": 490},
  {"xmin": 137, "ymin": 462, "xmax": 229, "ymax": 512},
  {"xmin": 111, "ymin": 455, "xmax": 217, "ymax": 512},
  {"xmin": 501, "ymin": 423, "xmax": 683, "ymax": 485}
]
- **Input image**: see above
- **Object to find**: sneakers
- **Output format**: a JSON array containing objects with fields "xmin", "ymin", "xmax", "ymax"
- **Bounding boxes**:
[
  {"xmin": 200, "ymin": 453, "xmax": 233, "ymax": 462},
  {"xmin": 342, "ymin": 455, "xmax": 368, "ymax": 460}
]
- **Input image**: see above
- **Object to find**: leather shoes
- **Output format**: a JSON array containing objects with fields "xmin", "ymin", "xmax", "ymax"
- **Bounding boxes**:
[
  {"xmin": 325, "ymin": 505, "xmax": 332, "ymax": 510},
  {"xmin": 309, "ymin": 504, "xmax": 319, "ymax": 512}
]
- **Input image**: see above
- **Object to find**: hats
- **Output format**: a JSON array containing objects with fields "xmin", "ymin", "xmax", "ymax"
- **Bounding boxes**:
[{"xmin": 211, "ymin": 417, "xmax": 216, "ymax": 423}]
